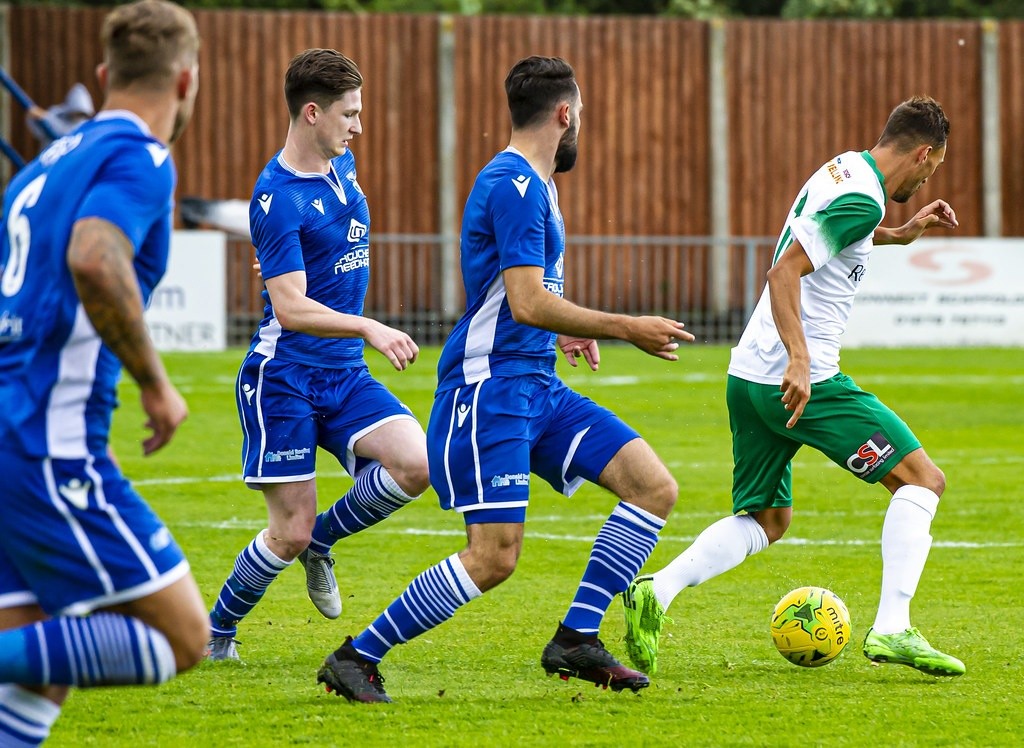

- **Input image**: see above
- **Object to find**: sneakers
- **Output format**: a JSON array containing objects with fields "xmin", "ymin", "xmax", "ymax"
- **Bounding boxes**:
[
  {"xmin": 206, "ymin": 633, "xmax": 242, "ymax": 664},
  {"xmin": 619, "ymin": 575, "xmax": 674, "ymax": 676},
  {"xmin": 315, "ymin": 635, "xmax": 393, "ymax": 705},
  {"xmin": 297, "ymin": 548, "xmax": 342, "ymax": 621},
  {"xmin": 539, "ymin": 619, "xmax": 651, "ymax": 695},
  {"xmin": 863, "ymin": 623, "xmax": 966, "ymax": 678}
]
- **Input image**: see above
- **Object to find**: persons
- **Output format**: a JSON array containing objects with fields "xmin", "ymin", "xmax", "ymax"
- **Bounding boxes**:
[
  {"xmin": 621, "ymin": 97, "xmax": 967, "ymax": 677},
  {"xmin": 319, "ymin": 56, "xmax": 695, "ymax": 704},
  {"xmin": 204, "ymin": 49, "xmax": 431, "ymax": 657},
  {"xmin": 26, "ymin": 82, "xmax": 93, "ymax": 151},
  {"xmin": 0, "ymin": 0, "xmax": 210, "ymax": 748}
]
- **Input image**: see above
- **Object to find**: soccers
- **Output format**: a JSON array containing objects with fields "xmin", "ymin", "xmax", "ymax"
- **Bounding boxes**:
[{"xmin": 770, "ymin": 586, "xmax": 852, "ymax": 668}]
